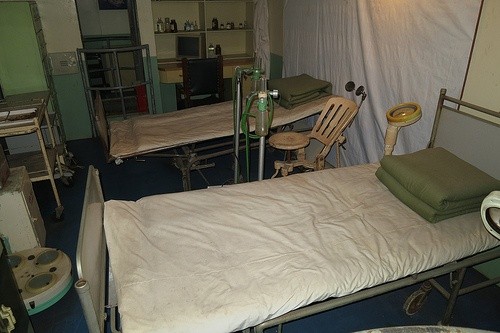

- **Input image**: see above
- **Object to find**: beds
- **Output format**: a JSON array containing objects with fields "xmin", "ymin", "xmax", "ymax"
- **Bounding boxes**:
[{"xmin": 75, "ymin": 87, "xmax": 500, "ymax": 333}]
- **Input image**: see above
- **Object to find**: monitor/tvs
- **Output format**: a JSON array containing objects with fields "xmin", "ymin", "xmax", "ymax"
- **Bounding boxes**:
[{"xmin": 175, "ymin": 35, "xmax": 202, "ymax": 60}]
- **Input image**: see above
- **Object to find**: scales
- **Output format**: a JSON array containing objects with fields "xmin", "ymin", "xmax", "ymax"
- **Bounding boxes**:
[{"xmin": 383, "ymin": 101, "xmax": 423, "ymax": 156}]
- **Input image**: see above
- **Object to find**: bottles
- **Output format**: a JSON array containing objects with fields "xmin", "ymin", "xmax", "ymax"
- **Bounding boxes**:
[
  {"xmin": 239, "ymin": 23, "xmax": 243, "ymax": 29},
  {"xmin": 184, "ymin": 19, "xmax": 198, "ymax": 32},
  {"xmin": 220, "ymin": 23, "xmax": 224, "ymax": 29},
  {"xmin": 157, "ymin": 17, "xmax": 164, "ymax": 33},
  {"xmin": 212, "ymin": 17, "xmax": 218, "ymax": 30},
  {"xmin": 163, "ymin": 18, "xmax": 170, "ymax": 33},
  {"xmin": 170, "ymin": 19, "xmax": 177, "ymax": 33},
  {"xmin": 231, "ymin": 22, "xmax": 234, "ymax": 29},
  {"xmin": 208, "ymin": 44, "xmax": 215, "ymax": 59},
  {"xmin": 250, "ymin": 68, "xmax": 261, "ymax": 98},
  {"xmin": 216, "ymin": 44, "xmax": 222, "ymax": 57},
  {"xmin": 244, "ymin": 21, "xmax": 248, "ymax": 29},
  {"xmin": 255, "ymin": 99, "xmax": 269, "ymax": 136},
  {"xmin": 226, "ymin": 22, "xmax": 231, "ymax": 29}
]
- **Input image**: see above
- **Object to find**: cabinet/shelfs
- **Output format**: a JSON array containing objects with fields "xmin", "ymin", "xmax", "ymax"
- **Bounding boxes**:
[
  {"xmin": 0, "ymin": 166, "xmax": 47, "ymax": 252},
  {"xmin": 150, "ymin": 0, "xmax": 255, "ymax": 101}
]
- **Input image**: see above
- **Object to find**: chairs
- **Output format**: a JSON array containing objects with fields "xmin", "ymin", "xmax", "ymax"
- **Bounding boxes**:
[
  {"xmin": 268, "ymin": 97, "xmax": 358, "ymax": 179},
  {"xmin": 106, "ymin": 91, "xmax": 334, "ymax": 192},
  {"xmin": 175, "ymin": 54, "xmax": 223, "ymax": 110}
]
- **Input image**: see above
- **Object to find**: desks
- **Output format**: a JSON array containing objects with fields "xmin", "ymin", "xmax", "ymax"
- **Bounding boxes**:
[{"xmin": 0, "ymin": 91, "xmax": 63, "ymax": 207}]
